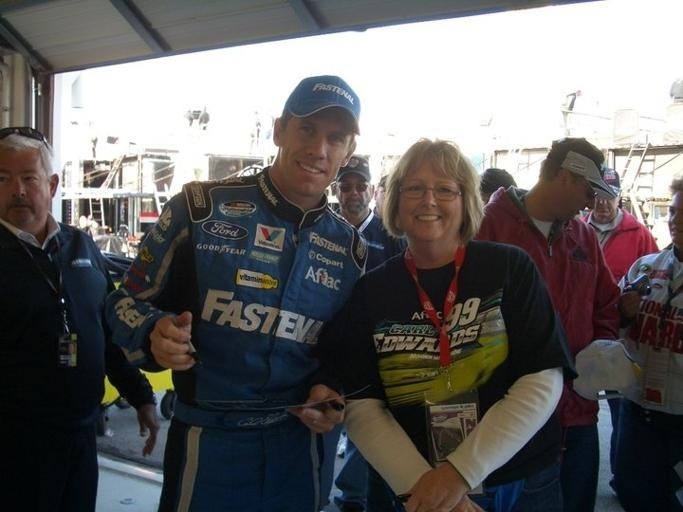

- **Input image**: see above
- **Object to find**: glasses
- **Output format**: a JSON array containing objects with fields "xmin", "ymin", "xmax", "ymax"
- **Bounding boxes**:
[
  {"xmin": 339, "ymin": 182, "xmax": 368, "ymax": 192},
  {"xmin": 0, "ymin": 126, "xmax": 49, "ymax": 149},
  {"xmin": 398, "ymin": 181, "xmax": 464, "ymax": 202}
]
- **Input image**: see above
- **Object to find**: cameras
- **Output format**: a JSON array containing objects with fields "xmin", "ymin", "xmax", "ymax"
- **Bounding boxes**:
[{"xmin": 622, "ymin": 275, "xmax": 651, "ymax": 296}]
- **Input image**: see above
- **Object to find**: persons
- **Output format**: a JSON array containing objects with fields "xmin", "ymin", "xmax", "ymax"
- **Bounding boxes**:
[
  {"xmin": 616, "ymin": 179, "xmax": 683, "ymax": 511},
  {"xmin": 105, "ymin": 75, "xmax": 368, "ymax": 512},
  {"xmin": 333, "ymin": 155, "xmax": 406, "ymax": 273},
  {"xmin": 372, "ymin": 176, "xmax": 388, "ymax": 218},
  {"xmin": 0, "ymin": 127, "xmax": 160, "ymax": 511},
  {"xmin": 322, "ymin": 141, "xmax": 580, "ymax": 512},
  {"xmin": 577, "ymin": 167, "xmax": 660, "ymax": 496},
  {"xmin": 480, "ymin": 168, "xmax": 517, "ymax": 207},
  {"xmin": 473, "ymin": 139, "xmax": 621, "ymax": 512}
]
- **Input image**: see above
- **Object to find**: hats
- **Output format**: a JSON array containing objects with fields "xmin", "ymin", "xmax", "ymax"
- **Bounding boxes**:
[
  {"xmin": 556, "ymin": 148, "xmax": 617, "ymax": 199},
  {"xmin": 336, "ymin": 155, "xmax": 371, "ymax": 182},
  {"xmin": 600, "ymin": 166, "xmax": 621, "ymax": 195},
  {"xmin": 284, "ymin": 75, "xmax": 360, "ymax": 136}
]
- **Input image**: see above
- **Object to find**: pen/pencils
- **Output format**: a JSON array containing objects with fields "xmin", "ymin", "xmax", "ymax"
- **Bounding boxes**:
[{"xmin": 171, "ymin": 314, "xmax": 203, "ymax": 367}]
